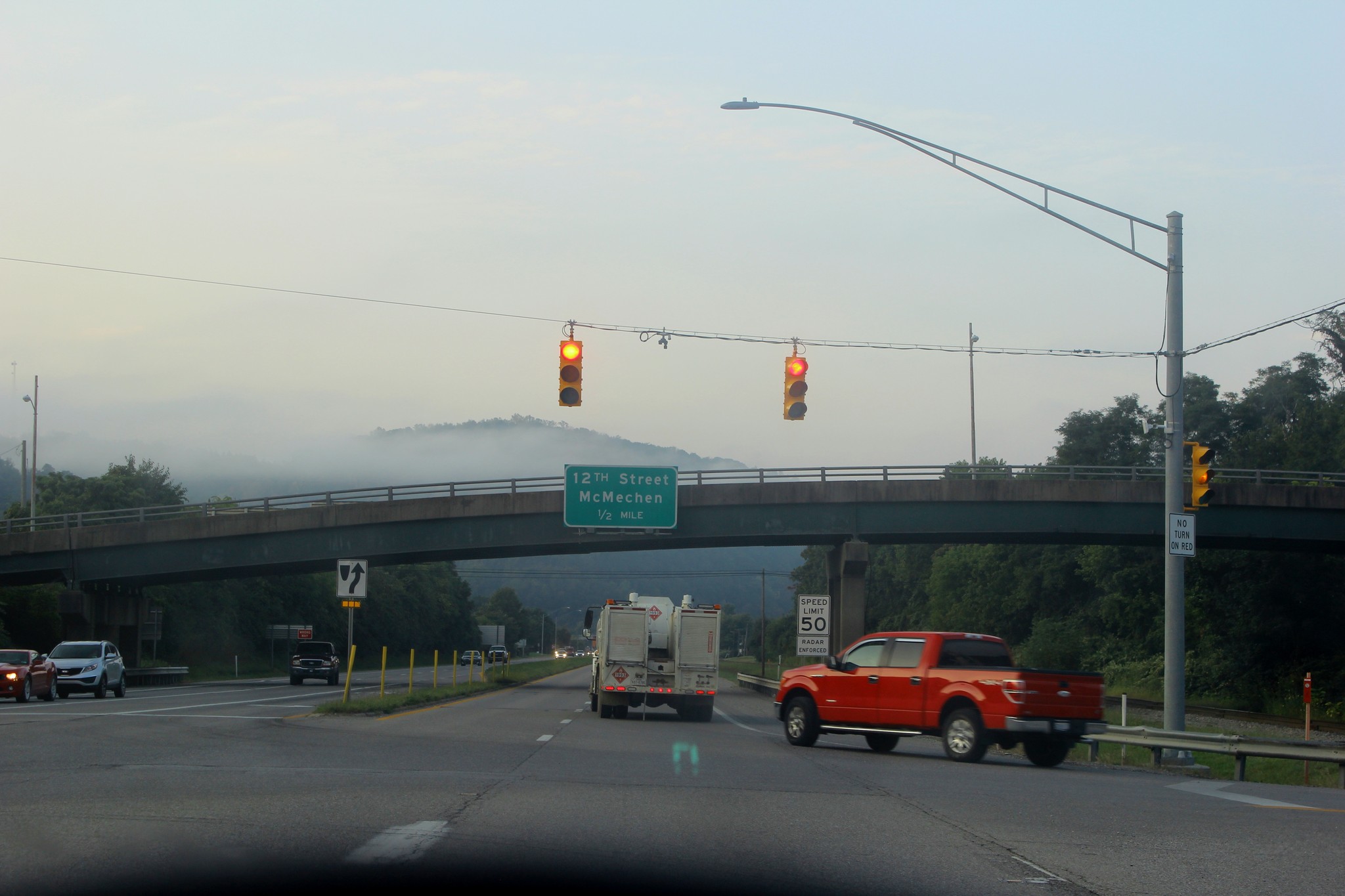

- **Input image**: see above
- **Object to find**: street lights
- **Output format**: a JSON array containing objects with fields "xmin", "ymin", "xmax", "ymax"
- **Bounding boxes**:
[{"xmin": 718, "ymin": 96, "xmax": 1192, "ymax": 739}]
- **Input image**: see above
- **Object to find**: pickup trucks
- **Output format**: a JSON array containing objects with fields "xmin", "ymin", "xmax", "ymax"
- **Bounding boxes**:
[{"xmin": 771, "ymin": 630, "xmax": 1107, "ymax": 769}]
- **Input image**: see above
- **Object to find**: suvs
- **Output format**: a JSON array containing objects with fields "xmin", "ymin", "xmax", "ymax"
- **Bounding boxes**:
[
  {"xmin": 488, "ymin": 645, "xmax": 509, "ymax": 663},
  {"xmin": 40, "ymin": 640, "xmax": 127, "ymax": 699},
  {"xmin": 289, "ymin": 642, "xmax": 341, "ymax": 685}
]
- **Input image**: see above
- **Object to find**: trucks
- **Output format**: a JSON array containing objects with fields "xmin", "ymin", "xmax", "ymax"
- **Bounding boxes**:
[{"xmin": 576, "ymin": 592, "xmax": 723, "ymax": 722}]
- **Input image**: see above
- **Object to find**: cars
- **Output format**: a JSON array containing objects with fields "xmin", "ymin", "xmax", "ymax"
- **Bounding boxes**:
[
  {"xmin": 0, "ymin": 648, "xmax": 58, "ymax": 702},
  {"xmin": 555, "ymin": 647, "xmax": 594, "ymax": 659},
  {"xmin": 461, "ymin": 650, "xmax": 483, "ymax": 666}
]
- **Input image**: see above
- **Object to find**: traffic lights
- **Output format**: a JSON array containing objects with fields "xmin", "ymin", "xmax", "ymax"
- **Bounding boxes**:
[
  {"xmin": 1191, "ymin": 445, "xmax": 1216, "ymax": 507},
  {"xmin": 559, "ymin": 340, "xmax": 584, "ymax": 406},
  {"xmin": 783, "ymin": 357, "xmax": 809, "ymax": 421}
]
